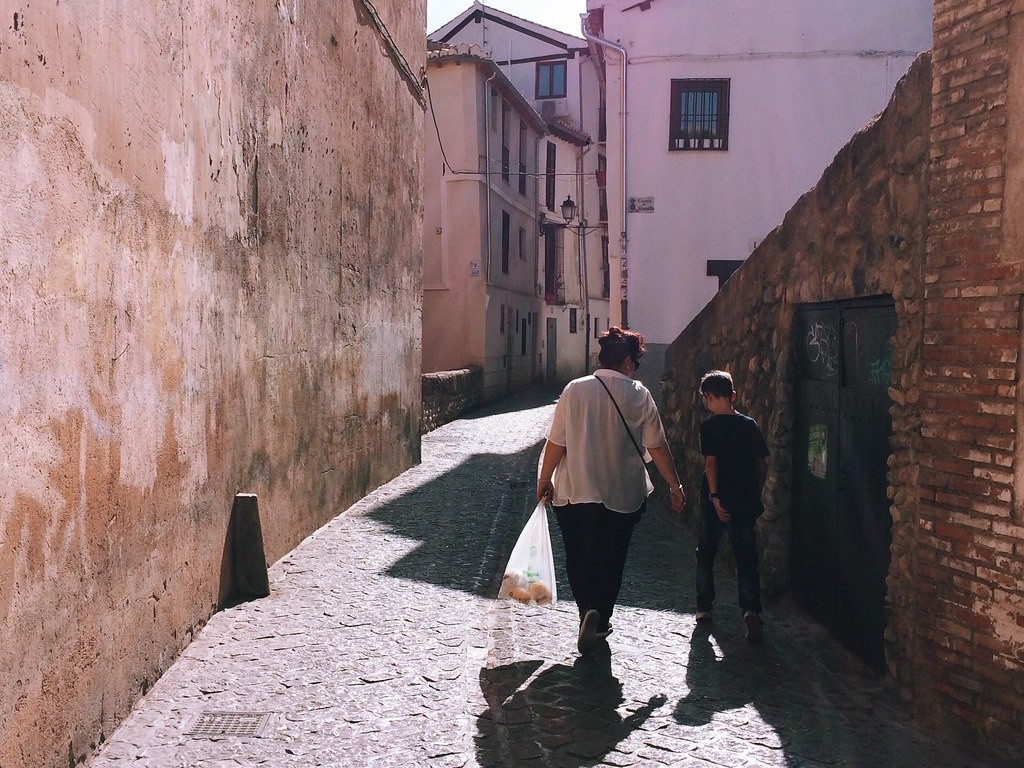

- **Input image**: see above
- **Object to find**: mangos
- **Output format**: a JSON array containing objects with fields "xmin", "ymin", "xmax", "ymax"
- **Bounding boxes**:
[{"xmin": 501, "ymin": 572, "xmax": 548, "ymax": 604}]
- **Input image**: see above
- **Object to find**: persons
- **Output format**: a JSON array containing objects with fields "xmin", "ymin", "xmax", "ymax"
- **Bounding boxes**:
[
  {"xmin": 537, "ymin": 327, "xmax": 688, "ymax": 654},
  {"xmin": 696, "ymin": 370, "xmax": 768, "ymax": 642}
]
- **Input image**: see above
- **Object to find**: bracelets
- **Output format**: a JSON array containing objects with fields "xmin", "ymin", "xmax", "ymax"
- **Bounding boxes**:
[
  {"xmin": 670, "ymin": 483, "xmax": 683, "ymax": 495},
  {"xmin": 708, "ymin": 491, "xmax": 718, "ymax": 501},
  {"xmin": 538, "ymin": 479, "xmax": 551, "ymax": 482}
]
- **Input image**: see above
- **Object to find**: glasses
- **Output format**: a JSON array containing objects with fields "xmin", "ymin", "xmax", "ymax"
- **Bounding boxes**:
[{"xmin": 631, "ymin": 358, "xmax": 642, "ymax": 370}]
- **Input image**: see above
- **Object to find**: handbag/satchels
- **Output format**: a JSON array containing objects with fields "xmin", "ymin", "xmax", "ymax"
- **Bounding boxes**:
[{"xmin": 495, "ymin": 497, "xmax": 557, "ymax": 608}]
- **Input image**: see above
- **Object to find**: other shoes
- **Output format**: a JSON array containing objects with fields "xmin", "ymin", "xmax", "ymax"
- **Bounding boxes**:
[
  {"xmin": 597, "ymin": 617, "xmax": 613, "ymax": 636},
  {"xmin": 578, "ymin": 607, "xmax": 597, "ymax": 655},
  {"xmin": 695, "ymin": 609, "xmax": 712, "ymax": 622},
  {"xmin": 744, "ymin": 610, "xmax": 763, "ymax": 642}
]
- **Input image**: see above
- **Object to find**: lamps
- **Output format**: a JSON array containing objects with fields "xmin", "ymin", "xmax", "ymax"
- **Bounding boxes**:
[{"xmin": 539, "ymin": 194, "xmax": 576, "ymax": 237}]
